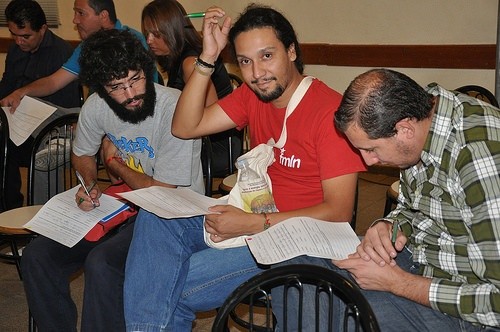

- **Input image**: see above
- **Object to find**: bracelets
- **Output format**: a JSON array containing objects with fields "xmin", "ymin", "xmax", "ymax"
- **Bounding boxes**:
[
  {"xmin": 193, "ymin": 55, "xmax": 215, "ymax": 77},
  {"xmin": 261, "ymin": 212, "xmax": 270, "ymax": 230},
  {"xmin": 106, "ymin": 156, "xmax": 115, "ymax": 167}
]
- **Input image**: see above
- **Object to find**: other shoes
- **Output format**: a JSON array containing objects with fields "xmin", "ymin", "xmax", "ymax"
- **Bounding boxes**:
[
  {"xmin": 5, "ymin": 245, "xmax": 26, "ymax": 264},
  {"xmin": 34, "ymin": 137, "xmax": 71, "ymax": 171}
]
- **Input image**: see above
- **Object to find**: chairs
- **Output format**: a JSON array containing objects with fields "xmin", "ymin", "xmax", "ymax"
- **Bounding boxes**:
[
  {"xmin": 0, "ymin": 113, "xmax": 100, "ymax": 332},
  {"xmin": 211, "ymin": 265, "xmax": 380, "ymax": 332},
  {"xmin": 203, "ymin": 73, "xmax": 248, "ymax": 198}
]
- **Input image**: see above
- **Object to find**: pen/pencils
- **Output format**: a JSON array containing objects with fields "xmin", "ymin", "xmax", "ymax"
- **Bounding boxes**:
[
  {"xmin": 391, "ymin": 218, "xmax": 398, "ymax": 246},
  {"xmin": 183, "ymin": 12, "xmax": 205, "ymax": 19},
  {"xmin": 77, "ymin": 178, "xmax": 98, "ymax": 206},
  {"xmin": 75, "ymin": 170, "xmax": 95, "ymax": 207}
]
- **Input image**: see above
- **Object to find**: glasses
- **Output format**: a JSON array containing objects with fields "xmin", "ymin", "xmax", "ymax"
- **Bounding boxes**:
[{"xmin": 104, "ymin": 73, "xmax": 146, "ymax": 97}]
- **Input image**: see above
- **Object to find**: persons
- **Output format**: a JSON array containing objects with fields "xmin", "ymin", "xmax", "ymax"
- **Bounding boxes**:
[
  {"xmin": 0, "ymin": 0, "xmax": 158, "ymax": 206},
  {"xmin": 271, "ymin": 69, "xmax": 500, "ymax": 332},
  {"xmin": 124, "ymin": 2, "xmax": 367, "ymax": 332},
  {"xmin": 19, "ymin": 28, "xmax": 205, "ymax": 332},
  {"xmin": 141, "ymin": 0, "xmax": 242, "ymax": 170},
  {"xmin": 0, "ymin": 0, "xmax": 84, "ymax": 215}
]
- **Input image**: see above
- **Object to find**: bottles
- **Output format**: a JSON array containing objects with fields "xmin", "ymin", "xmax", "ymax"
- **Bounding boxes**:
[{"xmin": 234, "ymin": 158, "xmax": 278, "ymax": 213}]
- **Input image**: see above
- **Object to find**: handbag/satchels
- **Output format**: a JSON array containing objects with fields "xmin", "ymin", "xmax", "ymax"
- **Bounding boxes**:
[
  {"xmin": 203, "ymin": 143, "xmax": 280, "ymax": 249},
  {"xmin": 83, "ymin": 178, "xmax": 139, "ymax": 245}
]
